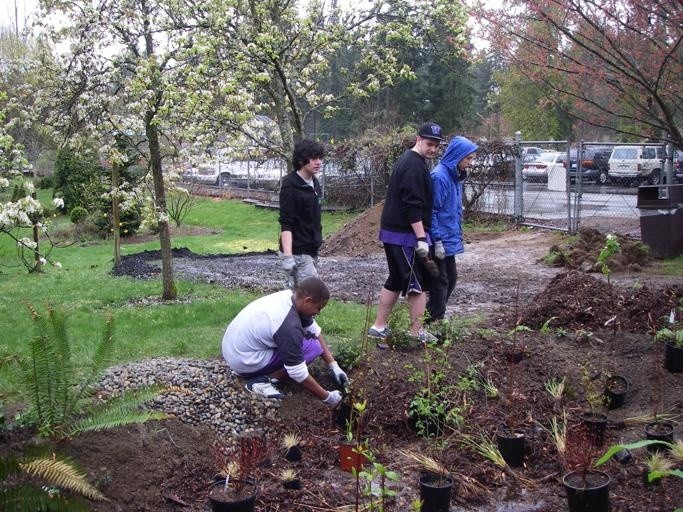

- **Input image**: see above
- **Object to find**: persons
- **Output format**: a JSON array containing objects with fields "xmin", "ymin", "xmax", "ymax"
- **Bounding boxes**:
[
  {"xmin": 367, "ymin": 121, "xmax": 438, "ymax": 345},
  {"xmin": 221, "ymin": 278, "xmax": 349, "ymax": 407},
  {"xmin": 278, "ymin": 138, "xmax": 325, "ymax": 288},
  {"xmin": 420, "ymin": 136, "xmax": 479, "ymax": 325}
]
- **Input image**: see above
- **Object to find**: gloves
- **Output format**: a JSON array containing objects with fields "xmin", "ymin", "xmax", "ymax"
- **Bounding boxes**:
[
  {"xmin": 328, "ymin": 360, "xmax": 350, "ymax": 385},
  {"xmin": 321, "ymin": 390, "xmax": 343, "ymax": 407},
  {"xmin": 434, "ymin": 239, "xmax": 446, "ymax": 260},
  {"xmin": 414, "ymin": 240, "xmax": 429, "ymax": 259},
  {"xmin": 282, "ymin": 254, "xmax": 298, "ymax": 277}
]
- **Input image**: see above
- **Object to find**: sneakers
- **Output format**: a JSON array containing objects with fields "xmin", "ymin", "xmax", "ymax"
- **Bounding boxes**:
[
  {"xmin": 367, "ymin": 325, "xmax": 393, "ymax": 339},
  {"xmin": 244, "ymin": 375, "xmax": 285, "ymax": 400},
  {"xmin": 416, "ymin": 327, "xmax": 440, "ymax": 343}
]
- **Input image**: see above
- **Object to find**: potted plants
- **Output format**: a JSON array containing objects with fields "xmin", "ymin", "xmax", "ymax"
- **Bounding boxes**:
[
  {"xmin": 278, "ymin": 464, "xmax": 302, "ymax": 491},
  {"xmin": 561, "ymin": 425, "xmax": 612, "ymax": 512},
  {"xmin": 203, "ymin": 434, "xmax": 258, "ymax": 511},
  {"xmin": 650, "ymin": 325, "xmax": 683, "ymax": 373},
  {"xmin": 278, "ymin": 427, "xmax": 307, "ymax": 461},
  {"xmin": 398, "ymin": 336, "xmax": 461, "ymax": 510}
]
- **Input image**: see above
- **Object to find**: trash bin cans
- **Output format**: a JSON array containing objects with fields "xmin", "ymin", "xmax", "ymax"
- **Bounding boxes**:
[{"xmin": 635, "ymin": 183, "xmax": 682, "ymax": 260}]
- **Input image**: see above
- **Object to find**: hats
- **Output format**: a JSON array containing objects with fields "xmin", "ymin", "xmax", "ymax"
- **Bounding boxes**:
[{"xmin": 420, "ymin": 122, "xmax": 443, "ymax": 141}]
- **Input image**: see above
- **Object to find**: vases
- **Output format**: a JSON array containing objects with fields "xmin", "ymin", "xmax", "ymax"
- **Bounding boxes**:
[
  {"xmin": 580, "ymin": 410, "xmax": 608, "ymax": 445},
  {"xmin": 335, "ymin": 394, "xmax": 367, "ymax": 431},
  {"xmin": 337, "ymin": 434, "xmax": 366, "ymax": 472},
  {"xmin": 642, "ymin": 421, "xmax": 676, "ymax": 454},
  {"xmin": 602, "ymin": 371, "xmax": 628, "ymax": 407},
  {"xmin": 408, "ymin": 396, "xmax": 446, "ymax": 438},
  {"xmin": 494, "ymin": 432, "xmax": 525, "ymax": 467}
]
